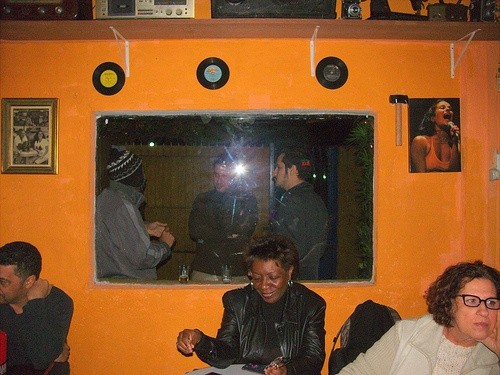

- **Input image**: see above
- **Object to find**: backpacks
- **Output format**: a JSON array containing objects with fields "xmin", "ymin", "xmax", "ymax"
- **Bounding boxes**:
[{"xmin": 328, "ymin": 299, "xmax": 403, "ymax": 375}]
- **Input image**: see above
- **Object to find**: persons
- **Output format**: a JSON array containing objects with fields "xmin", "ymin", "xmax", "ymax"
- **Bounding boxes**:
[
  {"xmin": 335, "ymin": 257, "xmax": 500, "ymax": 375},
  {"xmin": 411, "ymin": 97, "xmax": 461, "ymax": 172},
  {"xmin": 186, "ymin": 151, "xmax": 259, "ymax": 282},
  {"xmin": 1, "ymin": 241, "xmax": 75, "ymax": 375},
  {"xmin": 95, "ymin": 143, "xmax": 176, "ymax": 280},
  {"xmin": 177, "ymin": 234, "xmax": 327, "ymax": 375},
  {"xmin": 252, "ymin": 148, "xmax": 330, "ymax": 281},
  {"xmin": 13, "ymin": 108, "xmax": 52, "ymax": 167}
]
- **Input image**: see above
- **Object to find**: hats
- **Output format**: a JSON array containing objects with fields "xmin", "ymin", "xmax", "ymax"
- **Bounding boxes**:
[{"xmin": 107, "ymin": 148, "xmax": 145, "ymax": 188}]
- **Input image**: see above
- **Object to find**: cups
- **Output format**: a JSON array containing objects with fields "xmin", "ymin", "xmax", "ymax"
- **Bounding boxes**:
[
  {"xmin": 221, "ymin": 265, "xmax": 233, "ymax": 283},
  {"xmin": 177, "ymin": 265, "xmax": 193, "ymax": 282}
]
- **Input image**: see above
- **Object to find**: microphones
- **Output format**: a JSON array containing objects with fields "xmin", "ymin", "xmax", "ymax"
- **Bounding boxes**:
[{"xmin": 447, "ymin": 121, "xmax": 459, "ymax": 138}]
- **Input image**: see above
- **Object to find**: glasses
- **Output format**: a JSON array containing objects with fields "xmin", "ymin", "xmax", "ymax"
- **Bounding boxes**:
[{"xmin": 452, "ymin": 294, "xmax": 500, "ymax": 310}]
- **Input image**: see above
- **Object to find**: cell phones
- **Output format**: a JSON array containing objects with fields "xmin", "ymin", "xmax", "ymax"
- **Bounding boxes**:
[{"xmin": 242, "ymin": 362, "xmax": 267, "ymax": 374}]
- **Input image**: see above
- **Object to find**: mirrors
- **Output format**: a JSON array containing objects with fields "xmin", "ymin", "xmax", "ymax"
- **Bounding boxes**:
[{"xmin": 92, "ymin": 111, "xmax": 377, "ymax": 284}]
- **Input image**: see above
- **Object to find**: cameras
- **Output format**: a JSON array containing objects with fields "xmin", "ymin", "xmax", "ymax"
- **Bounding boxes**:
[{"xmin": 342, "ymin": 0, "xmax": 362, "ymax": 19}]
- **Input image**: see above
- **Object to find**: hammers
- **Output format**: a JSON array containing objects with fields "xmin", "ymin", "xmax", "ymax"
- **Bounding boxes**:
[{"xmin": 389, "ymin": 94, "xmax": 409, "ymax": 145}]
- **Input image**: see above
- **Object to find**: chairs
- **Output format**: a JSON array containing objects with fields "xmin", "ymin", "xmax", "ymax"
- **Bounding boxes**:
[{"xmin": 328, "ymin": 301, "xmax": 404, "ymax": 375}]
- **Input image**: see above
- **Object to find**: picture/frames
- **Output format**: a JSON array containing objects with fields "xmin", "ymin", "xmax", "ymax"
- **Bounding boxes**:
[{"xmin": 0, "ymin": 98, "xmax": 59, "ymax": 174}]
